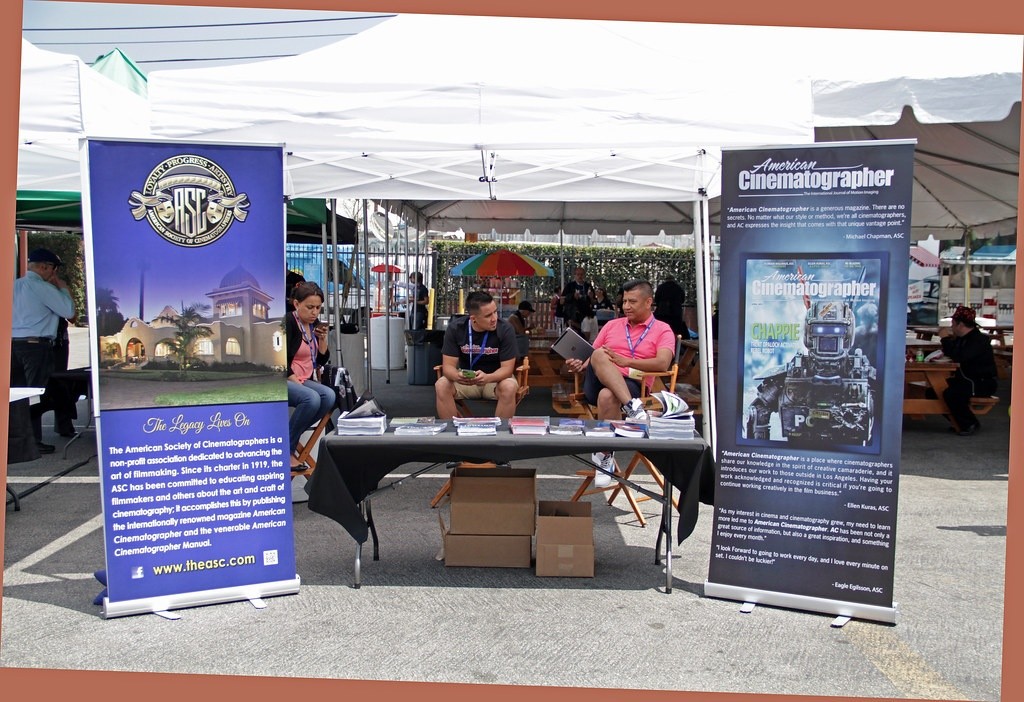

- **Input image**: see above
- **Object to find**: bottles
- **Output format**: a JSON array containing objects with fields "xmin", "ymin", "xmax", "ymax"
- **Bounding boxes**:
[{"xmin": 916, "ymin": 348, "xmax": 925, "ymax": 361}]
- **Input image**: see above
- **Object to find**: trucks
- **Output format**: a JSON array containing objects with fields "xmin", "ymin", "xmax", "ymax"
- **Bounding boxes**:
[{"xmin": 286, "ymin": 243, "xmax": 382, "ymax": 321}]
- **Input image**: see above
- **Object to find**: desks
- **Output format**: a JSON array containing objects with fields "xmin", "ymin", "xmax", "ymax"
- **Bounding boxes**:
[
  {"xmin": 682, "ymin": 338, "xmax": 718, "ymax": 386},
  {"xmin": 307, "ymin": 418, "xmax": 717, "ymax": 589},
  {"xmin": 984, "ymin": 326, "xmax": 1014, "ymax": 334},
  {"xmin": 914, "ymin": 327, "xmax": 940, "ymax": 341},
  {"xmin": 905, "ymin": 337, "xmax": 942, "ymax": 354},
  {"xmin": 982, "ymin": 334, "xmax": 1009, "ymax": 345},
  {"xmin": 902, "ymin": 362, "xmax": 961, "ymax": 434}
]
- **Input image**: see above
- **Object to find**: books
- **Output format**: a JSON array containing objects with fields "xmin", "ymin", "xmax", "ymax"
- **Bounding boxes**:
[{"xmin": 338, "ymin": 391, "xmax": 696, "ymax": 442}]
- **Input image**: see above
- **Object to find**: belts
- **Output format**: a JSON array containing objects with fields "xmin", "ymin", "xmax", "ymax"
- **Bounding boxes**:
[{"xmin": 12, "ymin": 337, "xmax": 53, "ymax": 344}]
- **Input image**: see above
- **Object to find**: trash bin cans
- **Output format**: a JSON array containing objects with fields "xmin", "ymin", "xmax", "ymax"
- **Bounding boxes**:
[{"xmin": 404, "ymin": 329, "xmax": 446, "ymax": 385}]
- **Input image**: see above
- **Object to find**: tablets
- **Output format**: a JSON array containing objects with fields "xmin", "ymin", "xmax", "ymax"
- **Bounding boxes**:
[{"xmin": 550, "ymin": 327, "xmax": 595, "ymax": 366}]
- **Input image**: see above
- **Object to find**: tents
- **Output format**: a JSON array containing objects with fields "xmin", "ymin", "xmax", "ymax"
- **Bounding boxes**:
[
  {"xmin": 814, "ymin": 28, "xmax": 1023, "ymax": 308},
  {"xmin": 16, "ymin": 191, "xmax": 362, "ymax": 339},
  {"xmin": 148, "ymin": 13, "xmax": 814, "ymax": 445},
  {"xmin": 938, "ymin": 257, "xmax": 1016, "ymax": 324},
  {"xmin": 14, "ymin": 35, "xmax": 149, "ymax": 195}
]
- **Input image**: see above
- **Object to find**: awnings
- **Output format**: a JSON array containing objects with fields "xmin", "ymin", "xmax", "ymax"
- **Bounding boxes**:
[{"xmin": 908, "ymin": 247, "xmax": 944, "ymax": 267}]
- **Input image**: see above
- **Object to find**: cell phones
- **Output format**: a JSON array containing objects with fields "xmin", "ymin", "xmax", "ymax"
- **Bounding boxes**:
[
  {"xmin": 314, "ymin": 320, "xmax": 329, "ymax": 332},
  {"xmin": 462, "ymin": 369, "xmax": 476, "ymax": 379}
]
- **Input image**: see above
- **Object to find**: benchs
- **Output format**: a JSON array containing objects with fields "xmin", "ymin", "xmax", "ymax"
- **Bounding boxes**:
[
  {"xmin": 968, "ymin": 395, "xmax": 1001, "ymax": 415},
  {"xmin": 993, "ymin": 349, "xmax": 1013, "ymax": 364}
]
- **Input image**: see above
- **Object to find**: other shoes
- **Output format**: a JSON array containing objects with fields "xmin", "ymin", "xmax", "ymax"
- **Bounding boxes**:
[
  {"xmin": 59, "ymin": 430, "xmax": 79, "ymax": 438},
  {"xmin": 446, "ymin": 461, "xmax": 462, "ymax": 469},
  {"xmin": 497, "ymin": 461, "xmax": 511, "ymax": 469},
  {"xmin": 34, "ymin": 442, "xmax": 56, "ymax": 454},
  {"xmin": 291, "ymin": 463, "xmax": 308, "ymax": 472},
  {"xmin": 955, "ymin": 420, "xmax": 979, "ymax": 435}
]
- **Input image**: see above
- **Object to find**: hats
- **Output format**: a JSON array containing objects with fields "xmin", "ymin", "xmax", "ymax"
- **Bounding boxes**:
[
  {"xmin": 950, "ymin": 305, "xmax": 978, "ymax": 324},
  {"xmin": 518, "ymin": 300, "xmax": 535, "ymax": 312},
  {"xmin": 28, "ymin": 248, "xmax": 64, "ymax": 266}
]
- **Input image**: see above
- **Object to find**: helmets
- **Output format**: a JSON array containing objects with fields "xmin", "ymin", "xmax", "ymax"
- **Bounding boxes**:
[{"xmin": 797, "ymin": 265, "xmax": 866, "ymax": 366}]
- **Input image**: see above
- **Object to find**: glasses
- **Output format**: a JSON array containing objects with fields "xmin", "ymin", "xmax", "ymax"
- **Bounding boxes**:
[{"xmin": 45, "ymin": 262, "xmax": 57, "ymax": 270}]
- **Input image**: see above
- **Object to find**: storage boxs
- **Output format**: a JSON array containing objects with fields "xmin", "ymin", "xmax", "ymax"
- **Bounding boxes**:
[{"xmin": 441, "ymin": 468, "xmax": 596, "ymax": 577}]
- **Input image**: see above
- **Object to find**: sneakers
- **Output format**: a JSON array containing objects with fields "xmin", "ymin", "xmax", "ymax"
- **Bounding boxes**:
[
  {"xmin": 621, "ymin": 397, "xmax": 647, "ymax": 420},
  {"xmin": 594, "ymin": 451, "xmax": 615, "ymax": 487}
]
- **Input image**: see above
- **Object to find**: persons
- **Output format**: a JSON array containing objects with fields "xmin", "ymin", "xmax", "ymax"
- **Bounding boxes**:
[
  {"xmin": 286, "ymin": 262, "xmax": 306, "ymax": 312},
  {"xmin": 508, "ymin": 301, "xmax": 536, "ymax": 376},
  {"xmin": 409, "ymin": 272, "xmax": 429, "ymax": 330},
  {"xmin": 550, "ymin": 267, "xmax": 626, "ymax": 341},
  {"xmin": 285, "ymin": 281, "xmax": 336, "ymax": 472},
  {"xmin": 10, "ymin": 249, "xmax": 81, "ymax": 454},
  {"xmin": 435, "ymin": 291, "xmax": 518, "ymax": 470},
  {"xmin": 654, "ymin": 276, "xmax": 685, "ymax": 322},
  {"xmin": 941, "ymin": 305, "xmax": 999, "ymax": 437},
  {"xmin": 565, "ymin": 278, "xmax": 676, "ymax": 488},
  {"xmin": 473, "ymin": 276, "xmax": 523, "ymax": 289},
  {"xmin": 712, "ymin": 301, "xmax": 719, "ymax": 339}
]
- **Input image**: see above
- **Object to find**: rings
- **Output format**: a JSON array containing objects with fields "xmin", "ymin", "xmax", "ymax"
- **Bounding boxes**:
[{"xmin": 321, "ymin": 334, "xmax": 323, "ymax": 336}]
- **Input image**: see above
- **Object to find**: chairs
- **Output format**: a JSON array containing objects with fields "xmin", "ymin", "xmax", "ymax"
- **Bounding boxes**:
[
  {"xmin": 554, "ymin": 334, "xmax": 683, "ymax": 531},
  {"xmin": 433, "ymin": 332, "xmax": 529, "ymax": 511}
]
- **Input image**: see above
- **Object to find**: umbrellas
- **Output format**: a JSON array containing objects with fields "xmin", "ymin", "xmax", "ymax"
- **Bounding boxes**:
[{"xmin": 450, "ymin": 248, "xmax": 554, "ymax": 320}]
